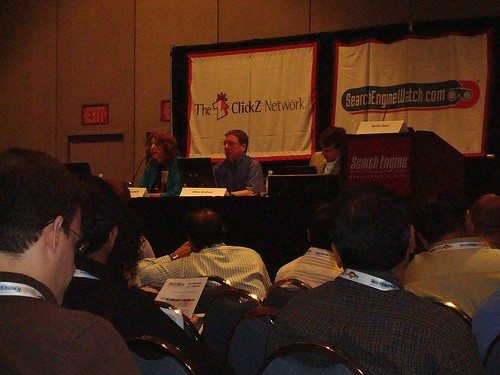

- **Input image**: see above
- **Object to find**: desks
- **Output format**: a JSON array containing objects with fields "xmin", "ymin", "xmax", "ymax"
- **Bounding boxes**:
[{"xmin": 120, "ymin": 196, "xmax": 269, "ymax": 259}]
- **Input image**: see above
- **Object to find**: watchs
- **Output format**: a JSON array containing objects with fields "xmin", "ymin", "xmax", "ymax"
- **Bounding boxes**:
[{"xmin": 170, "ymin": 251, "xmax": 179, "ymax": 261}]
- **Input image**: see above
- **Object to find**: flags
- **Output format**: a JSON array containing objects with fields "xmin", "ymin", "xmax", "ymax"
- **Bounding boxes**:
[
  {"xmin": 185, "ymin": 41, "xmax": 318, "ymax": 159},
  {"xmin": 332, "ymin": 29, "xmax": 491, "ymax": 155}
]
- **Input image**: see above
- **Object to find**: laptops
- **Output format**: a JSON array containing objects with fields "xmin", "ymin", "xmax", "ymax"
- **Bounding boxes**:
[
  {"xmin": 285, "ymin": 166, "xmax": 318, "ymax": 174},
  {"xmin": 176, "ymin": 157, "xmax": 218, "ymax": 187}
]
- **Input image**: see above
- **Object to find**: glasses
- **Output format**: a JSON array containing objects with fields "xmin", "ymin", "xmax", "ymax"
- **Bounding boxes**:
[{"xmin": 42, "ymin": 220, "xmax": 89, "ymax": 254}]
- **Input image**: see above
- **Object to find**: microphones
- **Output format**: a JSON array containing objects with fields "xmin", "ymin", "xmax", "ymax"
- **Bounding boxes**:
[{"xmin": 130, "ymin": 155, "xmax": 150, "ymax": 187}]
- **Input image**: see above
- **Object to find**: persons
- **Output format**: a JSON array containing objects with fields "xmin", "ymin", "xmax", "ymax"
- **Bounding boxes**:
[
  {"xmin": 317, "ymin": 127, "xmax": 348, "ymax": 175},
  {"xmin": 0, "ymin": 146, "xmax": 500, "ymax": 375},
  {"xmin": 137, "ymin": 132, "xmax": 185, "ymax": 198},
  {"xmin": 211, "ymin": 130, "xmax": 264, "ymax": 196}
]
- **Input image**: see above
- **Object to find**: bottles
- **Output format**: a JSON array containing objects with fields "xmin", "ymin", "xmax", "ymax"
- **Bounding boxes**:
[{"xmin": 266, "ymin": 170, "xmax": 272, "ymax": 193}]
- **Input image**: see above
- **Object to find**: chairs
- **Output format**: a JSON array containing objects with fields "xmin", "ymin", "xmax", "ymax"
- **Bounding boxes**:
[{"xmin": 123, "ymin": 276, "xmax": 365, "ymax": 375}]
